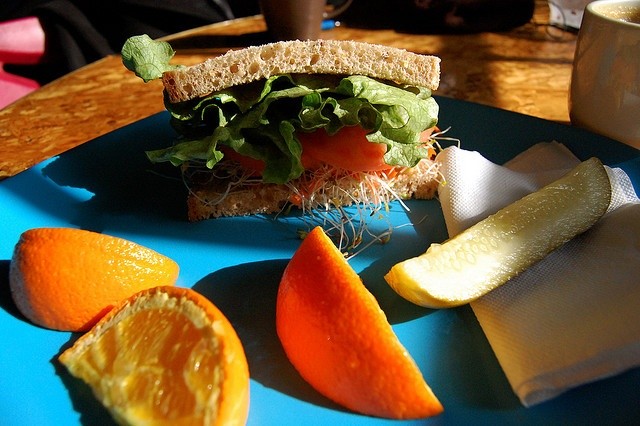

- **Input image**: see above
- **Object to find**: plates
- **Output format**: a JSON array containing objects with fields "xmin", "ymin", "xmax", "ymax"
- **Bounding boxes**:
[{"xmin": 1, "ymin": 94, "xmax": 639, "ymax": 426}]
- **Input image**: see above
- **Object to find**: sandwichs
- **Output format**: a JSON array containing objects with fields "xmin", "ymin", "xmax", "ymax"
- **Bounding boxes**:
[{"xmin": 144, "ymin": 38, "xmax": 445, "ymax": 223}]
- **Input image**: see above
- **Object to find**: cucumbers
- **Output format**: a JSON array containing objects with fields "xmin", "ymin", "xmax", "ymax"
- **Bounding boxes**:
[{"xmin": 388, "ymin": 153, "xmax": 612, "ymax": 305}]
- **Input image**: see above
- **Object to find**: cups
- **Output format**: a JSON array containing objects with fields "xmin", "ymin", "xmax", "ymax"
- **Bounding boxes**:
[
  {"xmin": 263, "ymin": 0, "xmax": 324, "ymax": 41},
  {"xmin": 568, "ymin": 1, "xmax": 640, "ymax": 148}
]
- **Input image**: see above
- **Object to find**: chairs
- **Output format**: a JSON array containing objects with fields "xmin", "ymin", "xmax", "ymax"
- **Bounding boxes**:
[{"xmin": 0, "ymin": 16, "xmax": 46, "ymax": 113}]
